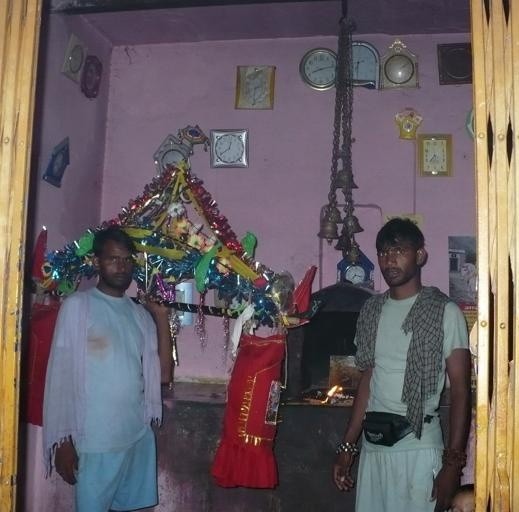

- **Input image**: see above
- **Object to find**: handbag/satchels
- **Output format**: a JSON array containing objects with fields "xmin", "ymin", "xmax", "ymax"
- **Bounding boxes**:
[{"xmin": 360, "ymin": 411, "xmax": 434, "ymax": 446}]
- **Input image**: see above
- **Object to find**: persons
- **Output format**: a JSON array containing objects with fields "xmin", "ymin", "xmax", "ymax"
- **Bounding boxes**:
[
  {"xmin": 448, "ymin": 482, "xmax": 490, "ymax": 511},
  {"xmin": 332, "ymin": 217, "xmax": 471, "ymax": 512},
  {"xmin": 41, "ymin": 224, "xmax": 175, "ymax": 511}
]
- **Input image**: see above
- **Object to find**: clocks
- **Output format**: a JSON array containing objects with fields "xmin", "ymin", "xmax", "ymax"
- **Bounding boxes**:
[
  {"xmin": 297, "ymin": 38, "xmax": 420, "ymax": 92},
  {"xmin": 153, "ymin": 61, "xmax": 276, "ymax": 176},
  {"xmin": 60, "ymin": 33, "xmax": 104, "ymax": 101},
  {"xmin": 42, "ymin": 136, "xmax": 70, "ymax": 189},
  {"xmin": 395, "ymin": 109, "xmax": 452, "ymax": 179},
  {"xmin": 337, "ymin": 247, "xmax": 376, "ymax": 288}
]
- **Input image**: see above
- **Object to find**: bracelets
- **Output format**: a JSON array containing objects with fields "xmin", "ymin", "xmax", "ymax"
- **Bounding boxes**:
[
  {"xmin": 336, "ymin": 441, "xmax": 359, "ymax": 456},
  {"xmin": 442, "ymin": 448, "xmax": 468, "ymax": 476}
]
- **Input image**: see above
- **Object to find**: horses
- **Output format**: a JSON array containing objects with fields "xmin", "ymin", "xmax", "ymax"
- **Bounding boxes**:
[{"xmin": 459, "ymin": 261, "xmax": 477, "ymax": 304}]
